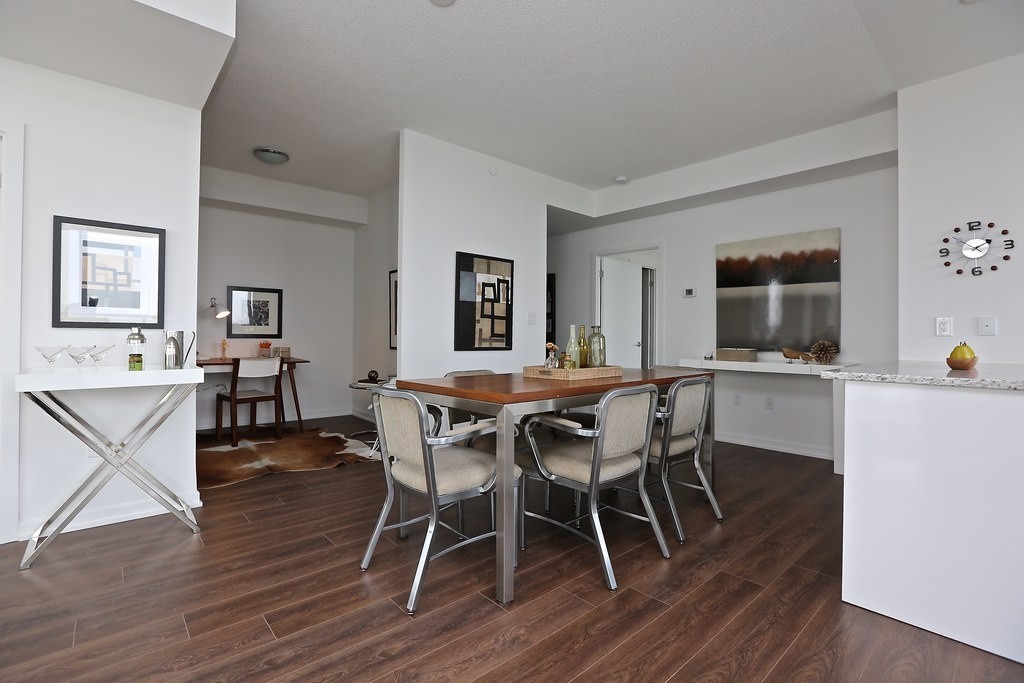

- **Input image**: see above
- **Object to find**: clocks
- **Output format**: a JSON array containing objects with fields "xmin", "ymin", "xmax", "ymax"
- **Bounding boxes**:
[{"xmin": 939, "ymin": 220, "xmax": 1014, "ymax": 277}]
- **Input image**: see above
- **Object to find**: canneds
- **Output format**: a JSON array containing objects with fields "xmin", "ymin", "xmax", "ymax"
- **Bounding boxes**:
[{"xmin": 128, "ymin": 354, "xmax": 143, "ymax": 371}]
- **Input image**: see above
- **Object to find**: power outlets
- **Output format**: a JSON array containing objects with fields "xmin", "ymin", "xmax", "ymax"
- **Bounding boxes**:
[{"xmin": 936, "ymin": 317, "xmax": 952, "ymax": 337}]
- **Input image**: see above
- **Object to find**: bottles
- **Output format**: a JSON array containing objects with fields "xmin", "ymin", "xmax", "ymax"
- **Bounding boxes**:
[
  {"xmin": 557, "ymin": 325, "xmax": 606, "ymax": 370},
  {"xmin": 126, "ymin": 326, "xmax": 148, "ymax": 372}
]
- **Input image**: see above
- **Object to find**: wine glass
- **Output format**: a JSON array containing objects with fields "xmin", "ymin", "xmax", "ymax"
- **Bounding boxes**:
[{"xmin": 34, "ymin": 346, "xmax": 108, "ymax": 371}]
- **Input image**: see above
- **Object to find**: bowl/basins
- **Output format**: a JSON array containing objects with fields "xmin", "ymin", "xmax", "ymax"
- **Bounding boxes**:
[{"xmin": 946, "ymin": 356, "xmax": 979, "ymax": 370}]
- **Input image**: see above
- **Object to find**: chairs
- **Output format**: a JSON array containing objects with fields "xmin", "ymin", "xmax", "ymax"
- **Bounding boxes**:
[
  {"xmin": 359, "ymin": 370, "xmax": 724, "ymax": 615},
  {"xmin": 216, "ymin": 357, "xmax": 285, "ymax": 447}
]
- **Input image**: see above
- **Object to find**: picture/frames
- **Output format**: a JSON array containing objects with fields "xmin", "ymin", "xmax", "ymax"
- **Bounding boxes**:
[
  {"xmin": 454, "ymin": 252, "xmax": 514, "ymax": 351},
  {"xmin": 51, "ymin": 215, "xmax": 165, "ymax": 329},
  {"xmin": 389, "ymin": 269, "xmax": 398, "ymax": 349},
  {"xmin": 226, "ymin": 286, "xmax": 283, "ymax": 339}
]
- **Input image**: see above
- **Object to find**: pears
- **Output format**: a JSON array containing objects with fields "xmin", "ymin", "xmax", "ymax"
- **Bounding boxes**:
[{"xmin": 950, "ymin": 341, "xmax": 976, "ymax": 360}]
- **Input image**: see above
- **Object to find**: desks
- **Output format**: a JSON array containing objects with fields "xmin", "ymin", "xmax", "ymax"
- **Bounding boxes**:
[
  {"xmin": 395, "ymin": 367, "xmax": 716, "ymax": 605},
  {"xmin": 196, "ymin": 357, "xmax": 310, "ymax": 433},
  {"xmin": 14, "ymin": 364, "xmax": 204, "ymax": 571},
  {"xmin": 349, "ymin": 383, "xmax": 379, "ymax": 436},
  {"xmin": 679, "ymin": 359, "xmax": 859, "ymax": 475}
]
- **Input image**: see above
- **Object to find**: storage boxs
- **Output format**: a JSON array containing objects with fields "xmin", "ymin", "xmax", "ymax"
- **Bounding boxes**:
[{"xmin": 273, "ymin": 347, "xmax": 290, "ymax": 358}]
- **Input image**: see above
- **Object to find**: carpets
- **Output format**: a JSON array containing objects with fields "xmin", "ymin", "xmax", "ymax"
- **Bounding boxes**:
[{"xmin": 197, "ymin": 427, "xmax": 382, "ymax": 490}]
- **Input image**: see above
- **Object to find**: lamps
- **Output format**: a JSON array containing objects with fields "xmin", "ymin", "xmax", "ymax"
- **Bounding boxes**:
[
  {"xmin": 210, "ymin": 297, "xmax": 230, "ymax": 318},
  {"xmin": 253, "ymin": 148, "xmax": 289, "ymax": 164}
]
